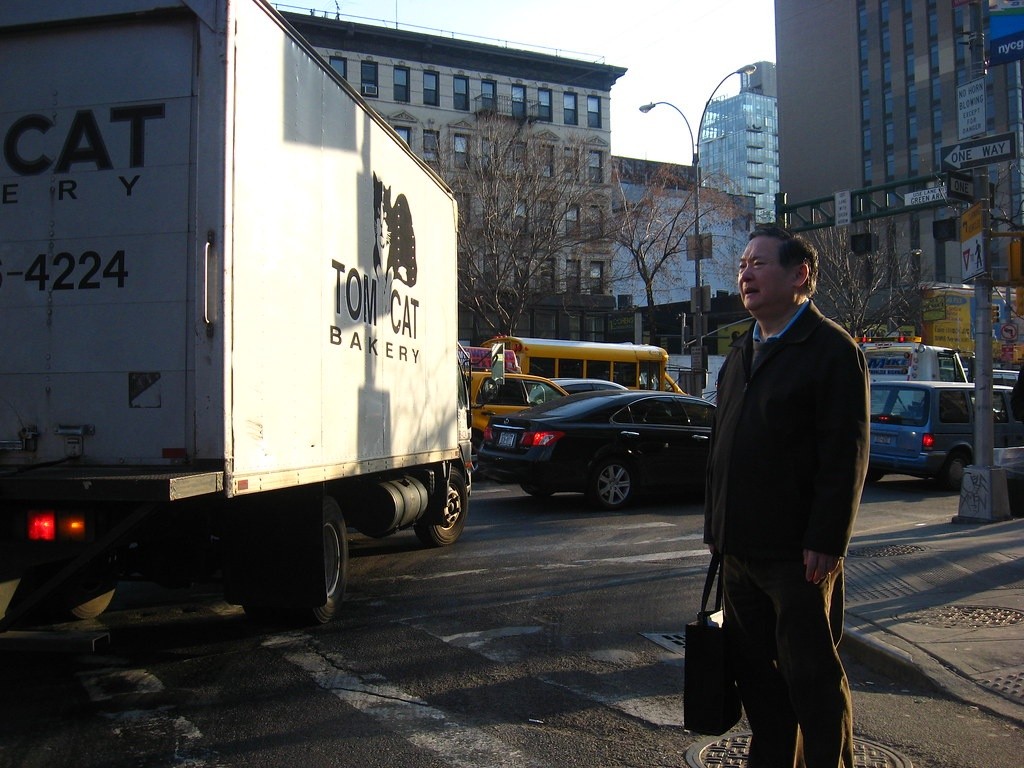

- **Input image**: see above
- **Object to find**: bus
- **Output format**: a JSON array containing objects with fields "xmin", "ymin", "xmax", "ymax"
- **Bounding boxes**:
[{"xmin": 477, "ymin": 336, "xmax": 694, "ymax": 396}]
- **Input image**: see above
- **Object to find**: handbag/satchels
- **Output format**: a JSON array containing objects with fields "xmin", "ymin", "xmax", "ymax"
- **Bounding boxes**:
[{"xmin": 684, "ymin": 554, "xmax": 746, "ymax": 739}]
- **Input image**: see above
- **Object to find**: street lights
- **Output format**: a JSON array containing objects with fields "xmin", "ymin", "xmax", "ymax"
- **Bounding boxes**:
[{"xmin": 638, "ymin": 65, "xmax": 758, "ymax": 400}]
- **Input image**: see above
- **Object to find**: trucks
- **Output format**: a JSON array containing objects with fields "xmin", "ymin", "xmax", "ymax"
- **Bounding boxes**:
[{"xmin": 1, "ymin": 0, "xmax": 505, "ymax": 626}]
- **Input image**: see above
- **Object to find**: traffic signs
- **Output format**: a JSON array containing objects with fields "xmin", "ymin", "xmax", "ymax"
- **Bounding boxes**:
[{"xmin": 937, "ymin": 132, "xmax": 1016, "ymax": 174}]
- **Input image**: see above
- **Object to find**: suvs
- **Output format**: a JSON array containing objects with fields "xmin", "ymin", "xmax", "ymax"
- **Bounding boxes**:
[
  {"xmin": 861, "ymin": 380, "xmax": 1023, "ymax": 493},
  {"xmin": 464, "ymin": 371, "xmax": 572, "ymax": 480}
]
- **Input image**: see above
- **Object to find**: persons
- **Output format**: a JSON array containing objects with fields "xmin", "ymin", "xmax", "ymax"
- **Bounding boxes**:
[{"xmin": 684, "ymin": 225, "xmax": 870, "ymax": 768}]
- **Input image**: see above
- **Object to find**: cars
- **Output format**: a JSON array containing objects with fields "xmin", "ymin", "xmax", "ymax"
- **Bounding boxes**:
[
  {"xmin": 526, "ymin": 377, "xmax": 631, "ymax": 409},
  {"xmin": 475, "ymin": 390, "xmax": 716, "ymax": 510}
]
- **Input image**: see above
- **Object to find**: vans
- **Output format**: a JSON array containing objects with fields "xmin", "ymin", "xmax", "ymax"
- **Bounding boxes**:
[
  {"xmin": 852, "ymin": 335, "xmax": 1000, "ymax": 418},
  {"xmin": 938, "ymin": 367, "xmax": 1021, "ymax": 386}
]
